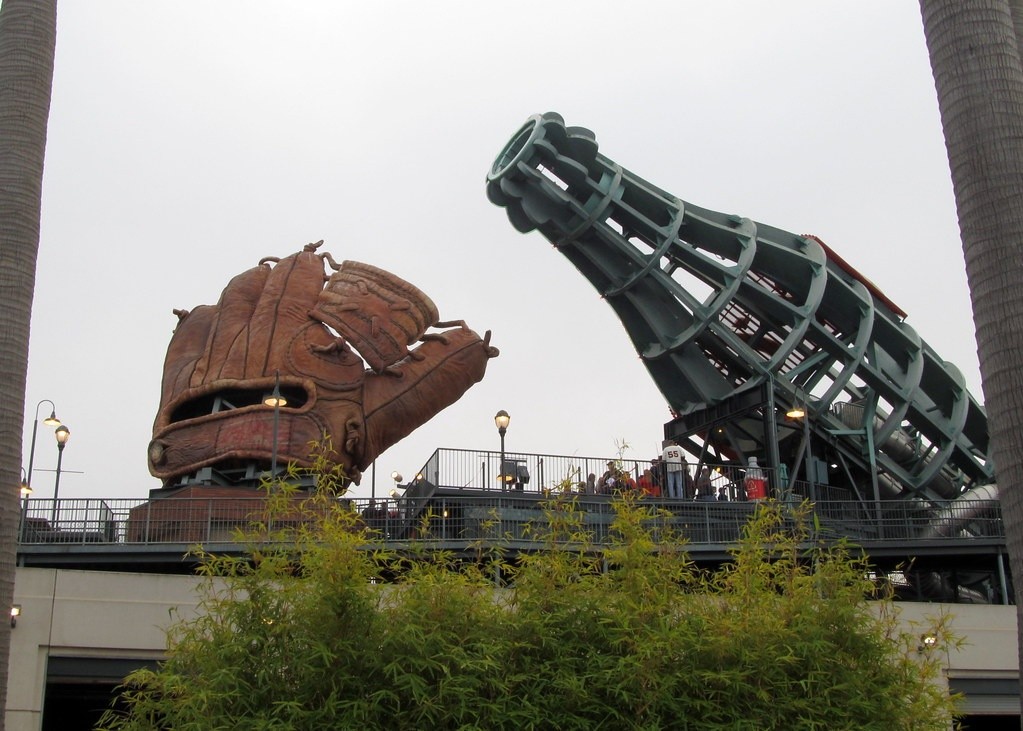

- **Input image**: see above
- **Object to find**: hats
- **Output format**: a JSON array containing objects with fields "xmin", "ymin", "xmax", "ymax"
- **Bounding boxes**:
[{"xmin": 607, "ymin": 462, "xmax": 613, "ymax": 465}]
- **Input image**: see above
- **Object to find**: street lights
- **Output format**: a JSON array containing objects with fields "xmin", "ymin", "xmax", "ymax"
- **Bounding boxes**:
[
  {"xmin": 17, "ymin": 397, "xmax": 60, "ymax": 545},
  {"xmin": 495, "ymin": 408, "xmax": 512, "ymax": 592},
  {"xmin": 50, "ymin": 424, "xmax": 71, "ymax": 530}
]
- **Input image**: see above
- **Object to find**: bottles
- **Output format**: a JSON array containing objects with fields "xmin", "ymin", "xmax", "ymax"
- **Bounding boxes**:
[{"xmin": 743, "ymin": 456, "xmax": 766, "ymax": 502}]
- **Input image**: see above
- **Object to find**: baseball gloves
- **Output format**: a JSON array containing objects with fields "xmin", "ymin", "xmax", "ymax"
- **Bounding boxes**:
[{"xmin": 145, "ymin": 235, "xmax": 500, "ymax": 483}]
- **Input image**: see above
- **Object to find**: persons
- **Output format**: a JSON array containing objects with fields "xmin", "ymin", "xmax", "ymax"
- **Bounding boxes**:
[
  {"xmin": 378, "ymin": 499, "xmax": 392, "ymax": 536},
  {"xmin": 564, "ymin": 438, "xmax": 749, "ymax": 501},
  {"xmin": 361, "ymin": 497, "xmax": 381, "ymax": 538}
]
